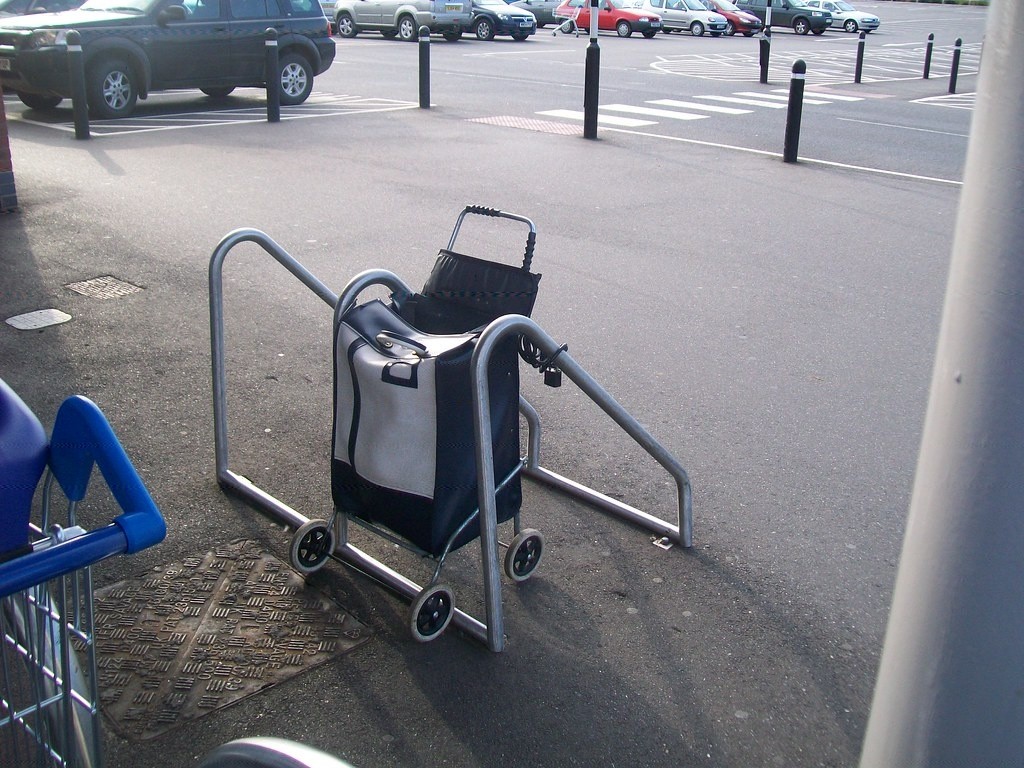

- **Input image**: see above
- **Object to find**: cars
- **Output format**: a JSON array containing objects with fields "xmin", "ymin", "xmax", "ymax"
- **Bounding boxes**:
[
  {"xmin": 469, "ymin": 0, "xmax": 537, "ymax": 41},
  {"xmin": 507, "ymin": 0, "xmax": 567, "ymax": 28},
  {"xmin": 555, "ymin": 0, "xmax": 665, "ymax": 39},
  {"xmin": 702, "ymin": 0, "xmax": 762, "ymax": 37},
  {"xmin": 621, "ymin": 0, "xmax": 643, "ymax": 9},
  {"xmin": 643, "ymin": 0, "xmax": 729, "ymax": 36},
  {"xmin": 794, "ymin": 0, "xmax": 880, "ymax": 34}
]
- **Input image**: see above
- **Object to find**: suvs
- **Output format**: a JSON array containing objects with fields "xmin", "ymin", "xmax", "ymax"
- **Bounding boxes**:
[
  {"xmin": 733, "ymin": 0, "xmax": 833, "ymax": 36},
  {"xmin": 1, "ymin": 1, "xmax": 335, "ymax": 108},
  {"xmin": 334, "ymin": 1, "xmax": 474, "ymax": 43}
]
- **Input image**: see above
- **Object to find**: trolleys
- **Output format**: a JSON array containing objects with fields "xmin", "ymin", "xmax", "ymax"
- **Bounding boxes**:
[
  {"xmin": 0, "ymin": 382, "xmax": 166, "ymax": 766},
  {"xmin": 551, "ymin": 5, "xmax": 583, "ymax": 38}
]
